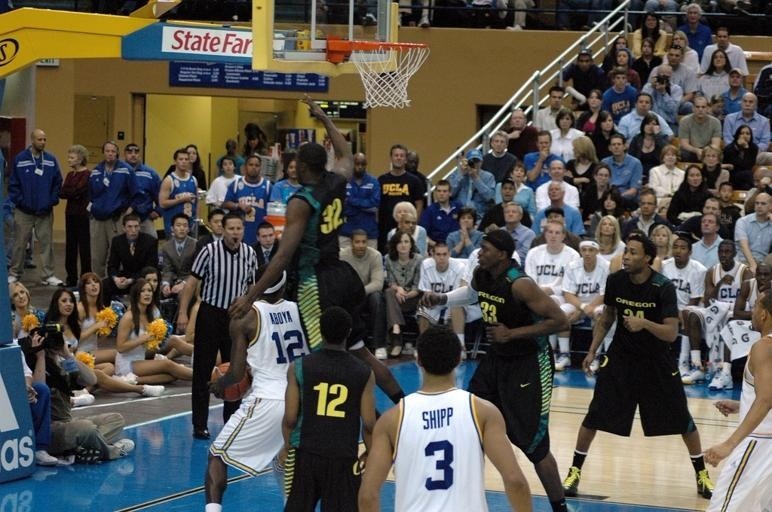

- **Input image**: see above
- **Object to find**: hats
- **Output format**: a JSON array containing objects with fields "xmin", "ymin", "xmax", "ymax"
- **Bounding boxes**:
[
  {"xmin": 729, "ymin": 68, "xmax": 742, "ymax": 76},
  {"xmin": 544, "ymin": 207, "xmax": 564, "ymax": 216},
  {"xmin": 466, "ymin": 150, "xmax": 481, "ymax": 161},
  {"xmin": 483, "ymin": 230, "xmax": 514, "ymax": 252}
]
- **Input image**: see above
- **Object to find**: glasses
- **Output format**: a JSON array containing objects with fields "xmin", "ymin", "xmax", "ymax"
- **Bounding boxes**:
[
  {"xmin": 127, "ymin": 145, "xmax": 139, "ymax": 152},
  {"xmin": 755, "ymin": 202, "xmax": 768, "ymax": 206},
  {"xmin": 641, "ymin": 202, "xmax": 655, "ymax": 205}
]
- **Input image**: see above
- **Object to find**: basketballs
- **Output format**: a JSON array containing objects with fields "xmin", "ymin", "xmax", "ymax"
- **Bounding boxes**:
[{"xmin": 211, "ymin": 361, "xmax": 251, "ymax": 402}]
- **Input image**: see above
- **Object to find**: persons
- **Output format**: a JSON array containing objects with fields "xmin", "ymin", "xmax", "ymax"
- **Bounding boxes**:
[
  {"xmin": 280, "ymin": 305, "xmax": 377, "ymax": 511},
  {"xmin": 413, "ymin": 229, "xmax": 571, "ymax": 512},
  {"xmin": 562, "ymin": 234, "xmax": 716, "ymax": 503},
  {"xmin": 701, "ymin": 291, "xmax": 771, "ymax": 511},
  {"xmin": 1, "ymin": 118, "xmax": 427, "ymax": 465},
  {"xmin": 356, "ymin": 324, "xmax": 532, "ymax": 512},
  {"xmin": 206, "ymin": 262, "xmax": 316, "ymax": 511},
  {"xmin": 304, "ymin": 0, "xmax": 771, "ymax": 388}
]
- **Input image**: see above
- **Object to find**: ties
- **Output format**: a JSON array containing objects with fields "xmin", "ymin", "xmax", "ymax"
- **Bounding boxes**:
[
  {"xmin": 129, "ymin": 243, "xmax": 135, "ymax": 255},
  {"xmin": 178, "ymin": 242, "xmax": 183, "ymax": 256},
  {"xmin": 263, "ymin": 251, "xmax": 271, "ymax": 264}
]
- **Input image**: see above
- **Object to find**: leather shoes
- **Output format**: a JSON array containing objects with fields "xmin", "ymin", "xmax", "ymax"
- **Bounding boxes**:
[
  {"xmin": 193, "ymin": 427, "xmax": 210, "ymax": 438},
  {"xmin": 390, "ymin": 346, "xmax": 403, "ymax": 359}
]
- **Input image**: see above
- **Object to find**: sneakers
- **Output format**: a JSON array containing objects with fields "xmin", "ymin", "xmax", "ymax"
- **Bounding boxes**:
[
  {"xmin": 563, "ymin": 466, "xmax": 581, "ymax": 496},
  {"xmin": 42, "ymin": 277, "xmax": 63, "ymax": 286},
  {"xmin": 8, "ymin": 276, "xmax": 19, "ymax": 284},
  {"xmin": 374, "ymin": 346, "xmax": 388, "ymax": 360},
  {"xmin": 554, "ymin": 356, "xmax": 601, "ymax": 373},
  {"xmin": 35, "ymin": 384, "xmax": 164, "ymax": 468},
  {"xmin": 695, "ymin": 469, "xmax": 715, "ymax": 501},
  {"xmin": 678, "ymin": 360, "xmax": 735, "ymax": 391}
]
